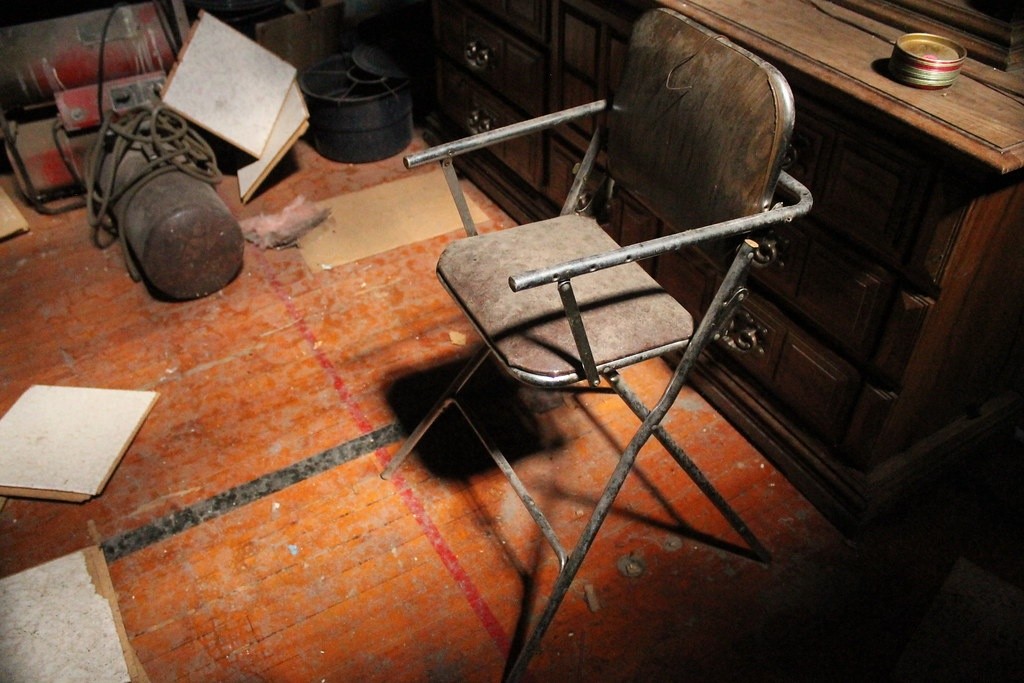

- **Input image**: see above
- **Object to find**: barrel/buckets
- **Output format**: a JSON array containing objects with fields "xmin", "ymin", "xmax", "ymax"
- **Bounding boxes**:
[{"xmin": 299, "ymin": 55, "xmax": 412, "ymax": 163}]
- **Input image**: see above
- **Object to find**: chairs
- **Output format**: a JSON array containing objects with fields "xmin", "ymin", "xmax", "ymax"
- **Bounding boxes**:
[{"xmin": 402, "ymin": 6, "xmax": 816, "ymax": 683}]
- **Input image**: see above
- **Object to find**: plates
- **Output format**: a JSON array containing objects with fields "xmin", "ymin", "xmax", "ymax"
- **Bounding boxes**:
[{"xmin": 889, "ymin": 33, "xmax": 967, "ymax": 90}]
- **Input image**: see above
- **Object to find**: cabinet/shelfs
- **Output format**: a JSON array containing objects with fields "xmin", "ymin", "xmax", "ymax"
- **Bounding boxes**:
[{"xmin": 403, "ymin": 0, "xmax": 1024, "ymax": 535}]
